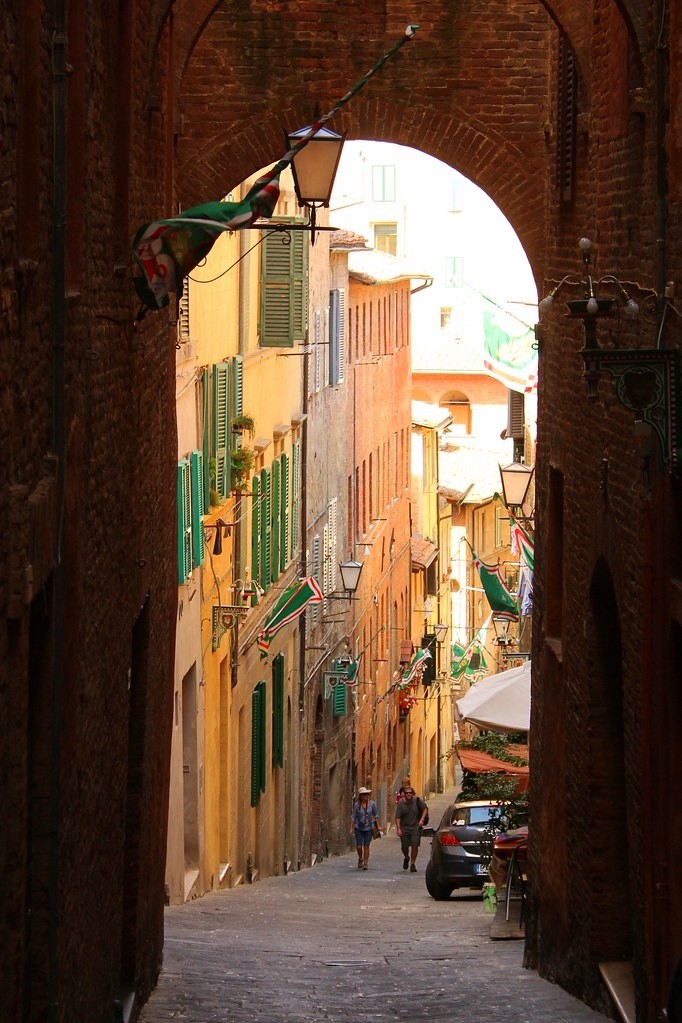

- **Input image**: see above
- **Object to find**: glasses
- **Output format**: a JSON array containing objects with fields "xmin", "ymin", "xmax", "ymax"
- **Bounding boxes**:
[{"xmin": 405, "ymin": 792, "xmax": 412, "ymax": 794}]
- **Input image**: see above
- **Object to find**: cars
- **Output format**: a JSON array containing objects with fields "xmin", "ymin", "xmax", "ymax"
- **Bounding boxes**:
[{"xmin": 419, "ymin": 799, "xmax": 529, "ymax": 901}]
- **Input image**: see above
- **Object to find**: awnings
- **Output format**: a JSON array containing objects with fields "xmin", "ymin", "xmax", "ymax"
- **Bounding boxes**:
[{"xmin": 455, "ymin": 661, "xmax": 529, "ymax": 793}]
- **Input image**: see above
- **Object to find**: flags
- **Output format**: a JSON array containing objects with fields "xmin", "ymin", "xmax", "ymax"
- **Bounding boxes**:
[
  {"xmin": 481, "ymin": 295, "xmax": 538, "ymax": 395},
  {"xmin": 130, "ymin": 43, "xmax": 408, "ymax": 310},
  {"xmin": 451, "ymin": 622, "xmax": 489, "ymax": 684},
  {"xmin": 257, "ymin": 577, "xmax": 325, "ymax": 654},
  {"xmin": 509, "ymin": 510, "xmax": 533, "ymax": 570},
  {"xmin": 468, "ymin": 545, "xmax": 519, "ymax": 623},
  {"xmin": 344, "ymin": 648, "xmax": 370, "ymax": 687},
  {"xmin": 397, "ymin": 649, "xmax": 432, "ymax": 688}
]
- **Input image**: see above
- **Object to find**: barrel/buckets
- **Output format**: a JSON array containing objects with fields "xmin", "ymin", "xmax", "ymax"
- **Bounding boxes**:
[{"xmin": 480, "ymin": 882, "xmax": 498, "ymax": 914}]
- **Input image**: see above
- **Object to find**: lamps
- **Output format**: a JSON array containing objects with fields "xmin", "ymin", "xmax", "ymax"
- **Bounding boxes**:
[
  {"xmin": 246, "ymin": 104, "xmax": 352, "ymax": 246},
  {"xmin": 332, "ymin": 552, "xmax": 365, "ymax": 605},
  {"xmin": 539, "ymin": 238, "xmax": 682, "ymax": 480},
  {"xmin": 492, "ymin": 617, "xmax": 522, "ymax": 646},
  {"xmin": 417, "ymin": 619, "xmax": 448, "ymax": 652}
]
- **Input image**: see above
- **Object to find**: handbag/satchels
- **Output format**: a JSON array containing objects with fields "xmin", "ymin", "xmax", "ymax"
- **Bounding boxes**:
[
  {"xmin": 417, "ymin": 797, "xmax": 429, "ymax": 825},
  {"xmin": 372, "ymin": 827, "xmax": 379, "ymax": 839}
]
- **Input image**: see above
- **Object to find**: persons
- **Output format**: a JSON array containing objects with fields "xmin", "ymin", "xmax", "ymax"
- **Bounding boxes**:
[
  {"xmin": 349, "ymin": 787, "xmax": 382, "ymax": 871},
  {"xmin": 396, "ymin": 777, "xmax": 428, "ymax": 872}
]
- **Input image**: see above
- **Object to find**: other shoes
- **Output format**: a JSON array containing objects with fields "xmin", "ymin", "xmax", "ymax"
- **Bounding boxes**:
[
  {"xmin": 363, "ymin": 864, "xmax": 368, "ymax": 870},
  {"xmin": 411, "ymin": 865, "xmax": 417, "ymax": 871},
  {"xmin": 358, "ymin": 858, "xmax": 363, "ymax": 868},
  {"xmin": 403, "ymin": 857, "xmax": 409, "ymax": 869}
]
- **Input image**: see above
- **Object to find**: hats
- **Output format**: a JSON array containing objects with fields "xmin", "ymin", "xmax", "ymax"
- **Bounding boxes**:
[{"xmin": 358, "ymin": 788, "xmax": 372, "ymax": 795}]
